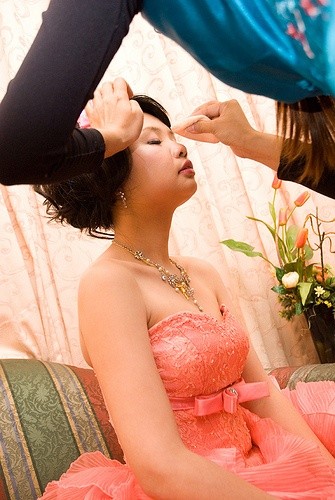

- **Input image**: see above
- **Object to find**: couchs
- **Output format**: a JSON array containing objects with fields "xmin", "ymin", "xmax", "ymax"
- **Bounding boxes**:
[{"xmin": 0, "ymin": 360, "xmax": 335, "ymax": 500}]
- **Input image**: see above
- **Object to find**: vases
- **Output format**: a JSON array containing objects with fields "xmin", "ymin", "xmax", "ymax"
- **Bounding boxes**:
[{"xmin": 300, "ymin": 305, "xmax": 335, "ymax": 364}]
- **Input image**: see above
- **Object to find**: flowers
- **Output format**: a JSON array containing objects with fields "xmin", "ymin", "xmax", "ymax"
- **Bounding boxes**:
[{"xmin": 217, "ymin": 171, "xmax": 335, "ymax": 334}]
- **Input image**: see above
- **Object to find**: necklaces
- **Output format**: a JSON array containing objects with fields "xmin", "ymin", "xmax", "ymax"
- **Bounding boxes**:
[{"xmin": 112, "ymin": 240, "xmax": 204, "ymax": 311}]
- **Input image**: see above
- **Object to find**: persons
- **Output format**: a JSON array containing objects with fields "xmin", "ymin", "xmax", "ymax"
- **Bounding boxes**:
[
  {"xmin": 35, "ymin": 94, "xmax": 335, "ymax": 500},
  {"xmin": 0, "ymin": 0, "xmax": 334, "ymax": 199}
]
